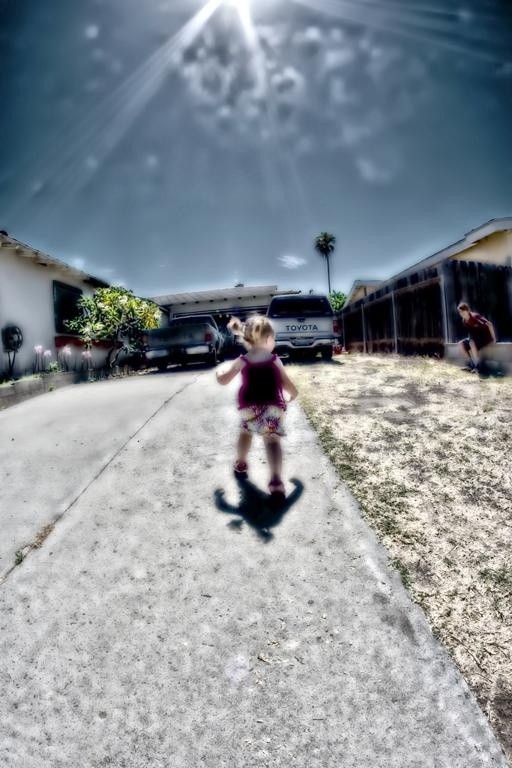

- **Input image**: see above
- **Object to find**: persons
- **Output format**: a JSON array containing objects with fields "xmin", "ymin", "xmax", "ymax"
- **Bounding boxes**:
[
  {"xmin": 219, "ymin": 314, "xmax": 298, "ymax": 495},
  {"xmin": 457, "ymin": 302, "xmax": 497, "ymax": 372}
]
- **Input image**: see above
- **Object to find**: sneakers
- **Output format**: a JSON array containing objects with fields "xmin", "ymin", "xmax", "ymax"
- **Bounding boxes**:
[
  {"xmin": 269, "ymin": 482, "xmax": 286, "ymax": 494},
  {"xmin": 233, "ymin": 460, "xmax": 247, "ymax": 473}
]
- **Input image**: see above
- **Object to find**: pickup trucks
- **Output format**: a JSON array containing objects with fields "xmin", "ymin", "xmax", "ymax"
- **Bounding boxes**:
[{"xmin": 141, "ymin": 314, "xmax": 225, "ymax": 372}]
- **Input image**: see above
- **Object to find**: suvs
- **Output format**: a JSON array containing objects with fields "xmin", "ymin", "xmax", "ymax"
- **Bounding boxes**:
[{"xmin": 266, "ymin": 294, "xmax": 338, "ymax": 360}]
